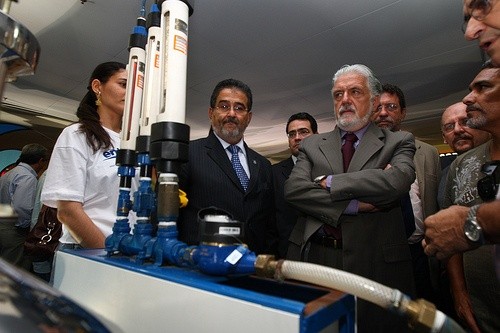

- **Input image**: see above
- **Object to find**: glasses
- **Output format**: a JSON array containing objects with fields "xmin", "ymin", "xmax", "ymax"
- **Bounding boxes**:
[
  {"xmin": 440, "ymin": 118, "xmax": 470, "ymax": 136},
  {"xmin": 210, "ymin": 104, "xmax": 248, "ymax": 116},
  {"xmin": 287, "ymin": 128, "xmax": 312, "ymax": 140},
  {"xmin": 376, "ymin": 102, "xmax": 401, "ymax": 114},
  {"xmin": 477, "ymin": 159, "xmax": 500, "ymax": 202}
]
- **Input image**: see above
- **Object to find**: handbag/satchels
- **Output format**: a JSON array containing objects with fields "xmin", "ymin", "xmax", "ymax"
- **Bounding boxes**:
[{"xmin": 24, "ymin": 200, "xmax": 63, "ymax": 254}]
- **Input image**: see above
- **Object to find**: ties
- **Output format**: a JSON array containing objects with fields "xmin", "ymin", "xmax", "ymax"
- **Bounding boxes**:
[
  {"xmin": 341, "ymin": 133, "xmax": 359, "ymax": 174},
  {"xmin": 227, "ymin": 144, "xmax": 251, "ymax": 192}
]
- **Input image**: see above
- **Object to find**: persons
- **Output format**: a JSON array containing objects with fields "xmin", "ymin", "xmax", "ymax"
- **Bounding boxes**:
[{"xmin": 0, "ymin": 0, "xmax": 500, "ymax": 333}]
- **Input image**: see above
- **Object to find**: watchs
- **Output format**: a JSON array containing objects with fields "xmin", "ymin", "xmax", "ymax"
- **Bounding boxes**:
[
  {"xmin": 464, "ymin": 204, "xmax": 484, "ymax": 247},
  {"xmin": 314, "ymin": 175, "xmax": 327, "ymax": 185}
]
